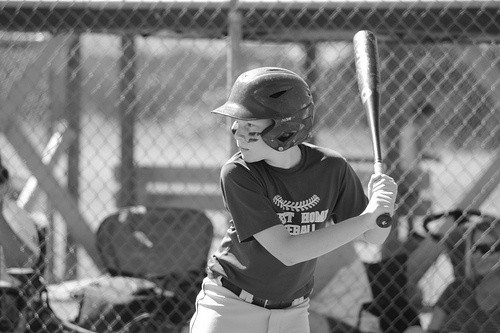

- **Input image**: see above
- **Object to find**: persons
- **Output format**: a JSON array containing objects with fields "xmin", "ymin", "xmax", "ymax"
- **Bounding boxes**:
[
  {"xmin": 328, "ymin": 263, "xmax": 421, "ymax": 333},
  {"xmin": 190, "ymin": 67, "xmax": 397, "ymax": 333},
  {"xmin": 0, "ymin": 193, "xmax": 41, "ymax": 333},
  {"xmin": 398, "ymin": 101, "xmax": 437, "ymax": 242},
  {"xmin": 426, "ymin": 222, "xmax": 500, "ymax": 333}
]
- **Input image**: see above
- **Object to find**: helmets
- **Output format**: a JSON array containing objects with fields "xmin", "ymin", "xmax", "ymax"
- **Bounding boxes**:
[{"xmin": 212, "ymin": 66, "xmax": 315, "ymax": 151}]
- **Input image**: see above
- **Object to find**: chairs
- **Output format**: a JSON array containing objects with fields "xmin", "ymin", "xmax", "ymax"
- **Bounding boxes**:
[{"xmin": 37, "ymin": 204, "xmax": 216, "ymax": 333}]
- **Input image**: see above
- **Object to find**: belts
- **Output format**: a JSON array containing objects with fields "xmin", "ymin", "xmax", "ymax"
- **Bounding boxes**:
[{"xmin": 208, "ymin": 269, "xmax": 310, "ymax": 309}]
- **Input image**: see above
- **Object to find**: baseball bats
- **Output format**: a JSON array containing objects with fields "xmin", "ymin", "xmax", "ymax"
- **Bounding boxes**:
[{"xmin": 353, "ymin": 30, "xmax": 394, "ymax": 228}]
